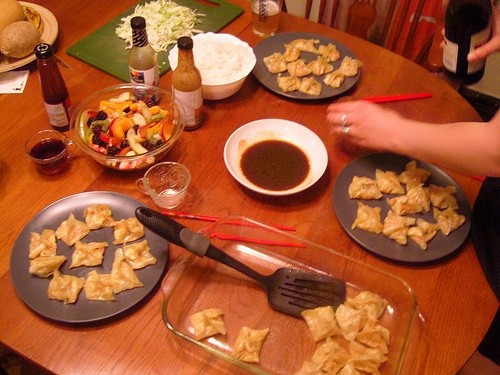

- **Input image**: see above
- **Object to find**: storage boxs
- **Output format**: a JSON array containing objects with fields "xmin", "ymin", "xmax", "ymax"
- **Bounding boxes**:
[{"xmin": 161, "ymin": 215, "xmax": 419, "ymax": 375}]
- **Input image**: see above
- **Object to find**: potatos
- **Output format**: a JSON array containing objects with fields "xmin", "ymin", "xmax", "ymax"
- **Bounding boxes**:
[{"xmin": 0, "ymin": 0, "xmax": 42, "ymax": 58}]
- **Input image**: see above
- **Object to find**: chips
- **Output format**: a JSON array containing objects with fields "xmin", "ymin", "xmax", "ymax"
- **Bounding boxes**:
[
  {"xmin": 190, "ymin": 308, "xmax": 227, "ymax": 340},
  {"xmin": 263, "ymin": 39, "xmax": 363, "ymax": 96},
  {"xmin": 29, "ymin": 205, "xmax": 156, "ymax": 302},
  {"xmin": 231, "ymin": 326, "xmax": 270, "ymax": 363},
  {"xmin": 296, "ymin": 291, "xmax": 390, "ymax": 374},
  {"xmin": 348, "ymin": 160, "xmax": 466, "ymax": 251}
]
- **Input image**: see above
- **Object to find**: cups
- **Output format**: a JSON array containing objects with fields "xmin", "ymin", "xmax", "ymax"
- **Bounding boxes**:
[
  {"xmin": 24, "ymin": 129, "xmax": 79, "ymax": 176},
  {"xmin": 251, "ymin": 0, "xmax": 283, "ymax": 38},
  {"xmin": 334, "ymin": 96, "xmax": 364, "ymax": 155},
  {"xmin": 135, "ymin": 162, "xmax": 191, "ymax": 210}
]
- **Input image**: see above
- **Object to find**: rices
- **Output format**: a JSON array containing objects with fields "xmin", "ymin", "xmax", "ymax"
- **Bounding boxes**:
[{"xmin": 193, "ymin": 37, "xmax": 252, "ymax": 85}]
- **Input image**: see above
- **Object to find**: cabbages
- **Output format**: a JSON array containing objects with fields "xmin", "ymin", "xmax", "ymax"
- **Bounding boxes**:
[{"xmin": 115, "ymin": 0, "xmax": 207, "ymax": 51}]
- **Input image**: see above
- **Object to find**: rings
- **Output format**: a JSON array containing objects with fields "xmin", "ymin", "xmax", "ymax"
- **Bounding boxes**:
[
  {"xmin": 344, "ymin": 128, "xmax": 349, "ymax": 136},
  {"xmin": 342, "ymin": 114, "xmax": 347, "ymax": 126}
]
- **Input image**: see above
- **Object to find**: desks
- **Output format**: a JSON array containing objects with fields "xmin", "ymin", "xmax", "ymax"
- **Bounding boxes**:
[{"xmin": 0, "ymin": 0, "xmax": 500, "ymax": 375}]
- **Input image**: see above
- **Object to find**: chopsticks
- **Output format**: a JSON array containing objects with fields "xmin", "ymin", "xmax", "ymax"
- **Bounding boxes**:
[{"xmin": 162, "ymin": 210, "xmax": 305, "ymax": 248}]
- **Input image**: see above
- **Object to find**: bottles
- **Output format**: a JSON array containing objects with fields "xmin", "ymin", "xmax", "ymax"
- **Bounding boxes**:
[
  {"xmin": 34, "ymin": 43, "xmax": 74, "ymax": 132},
  {"xmin": 442, "ymin": 0, "xmax": 492, "ymax": 85},
  {"xmin": 171, "ymin": 36, "xmax": 204, "ymax": 132},
  {"xmin": 128, "ymin": 16, "xmax": 160, "ymax": 107}
]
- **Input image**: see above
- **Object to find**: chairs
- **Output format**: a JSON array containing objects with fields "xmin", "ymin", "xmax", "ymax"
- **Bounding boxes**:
[{"xmin": 305, "ymin": 0, "xmax": 425, "ymax": 56}]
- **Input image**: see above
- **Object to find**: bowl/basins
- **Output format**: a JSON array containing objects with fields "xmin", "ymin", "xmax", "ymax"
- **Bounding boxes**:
[
  {"xmin": 168, "ymin": 32, "xmax": 257, "ymax": 101},
  {"xmin": 70, "ymin": 83, "xmax": 186, "ymax": 174}
]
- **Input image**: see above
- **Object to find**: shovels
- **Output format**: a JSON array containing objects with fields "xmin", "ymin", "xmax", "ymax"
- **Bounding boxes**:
[{"xmin": 135, "ymin": 207, "xmax": 346, "ymax": 319}]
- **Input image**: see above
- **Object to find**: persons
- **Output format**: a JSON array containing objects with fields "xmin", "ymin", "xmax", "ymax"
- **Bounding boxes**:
[{"xmin": 324, "ymin": 0, "xmax": 500, "ymax": 375}]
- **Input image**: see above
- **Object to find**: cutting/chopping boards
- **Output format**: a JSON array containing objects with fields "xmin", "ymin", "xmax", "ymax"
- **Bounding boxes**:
[{"xmin": 66, "ymin": 0, "xmax": 244, "ymax": 83}]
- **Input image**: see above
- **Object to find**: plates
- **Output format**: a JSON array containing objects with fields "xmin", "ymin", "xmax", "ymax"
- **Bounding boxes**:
[
  {"xmin": 223, "ymin": 118, "xmax": 328, "ymax": 196},
  {"xmin": 9, "ymin": 191, "xmax": 168, "ymax": 323},
  {"xmin": 161, "ymin": 215, "xmax": 426, "ymax": 375},
  {"xmin": 0, "ymin": 1, "xmax": 58, "ymax": 73},
  {"xmin": 333, "ymin": 152, "xmax": 471, "ymax": 263},
  {"xmin": 252, "ymin": 32, "xmax": 360, "ymax": 100}
]
